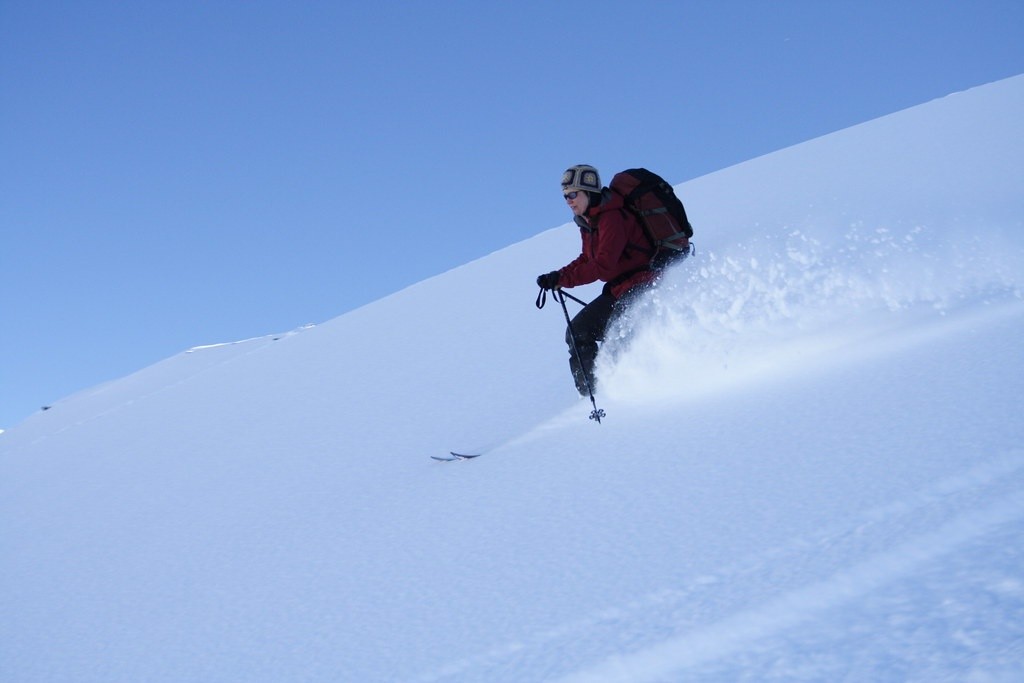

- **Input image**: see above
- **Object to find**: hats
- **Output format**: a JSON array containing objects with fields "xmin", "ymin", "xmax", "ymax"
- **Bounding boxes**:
[{"xmin": 562, "ymin": 165, "xmax": 601, "ymax": 194}]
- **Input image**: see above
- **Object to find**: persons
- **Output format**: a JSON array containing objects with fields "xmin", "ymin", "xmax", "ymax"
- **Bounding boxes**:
[{"xmin": 536, "ymin": 164, "xmax": 660, "ymax": 399}]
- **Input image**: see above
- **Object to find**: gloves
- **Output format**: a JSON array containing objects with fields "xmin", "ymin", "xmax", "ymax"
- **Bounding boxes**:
[{"xmin": 536, "ymin": 271, "xmax": 559, "ymax": 292}]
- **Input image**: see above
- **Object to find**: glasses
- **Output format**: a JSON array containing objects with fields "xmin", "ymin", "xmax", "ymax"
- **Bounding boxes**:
[{"xmin": 564, "ymin": 189, "xmax": 582, "ymax": 201}]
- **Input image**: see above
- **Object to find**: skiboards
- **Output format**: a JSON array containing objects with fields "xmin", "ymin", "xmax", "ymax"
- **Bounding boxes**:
[{"xmin": 431, "ymin": 448, "xmax": 477, "ymax": 462}]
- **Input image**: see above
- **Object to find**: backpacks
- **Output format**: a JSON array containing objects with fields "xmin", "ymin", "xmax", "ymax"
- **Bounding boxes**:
[{"xmin": 590, "ymin": 168, "xmax": 694, "ymax": 266}]
